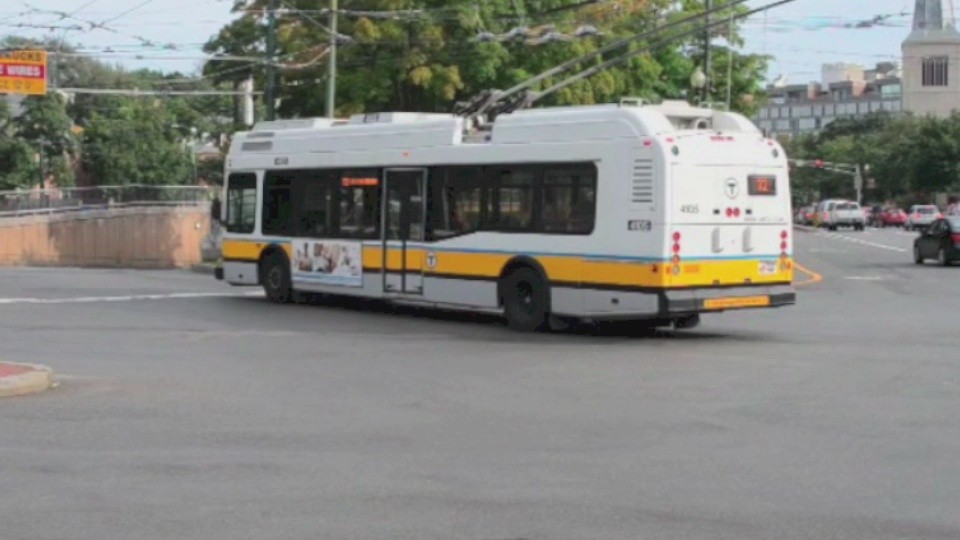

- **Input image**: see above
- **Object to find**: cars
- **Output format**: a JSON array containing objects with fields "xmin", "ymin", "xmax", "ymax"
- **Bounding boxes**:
[
  {"xmin": 797, "ymin": 199, "xmax": 867, "ymax": 230},
  {"xmin": 914, "ymin": 217, "xmax": 960, "ymax": 266},
  {"xmin": 863, "ymin": 203, "xmax": 939, "ymax": 230}
]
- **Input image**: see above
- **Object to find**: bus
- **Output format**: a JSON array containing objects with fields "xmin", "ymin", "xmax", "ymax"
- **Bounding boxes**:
[{"xmin": 211, "ymin": 99, "xmax": 792, "ymax": 336}]
